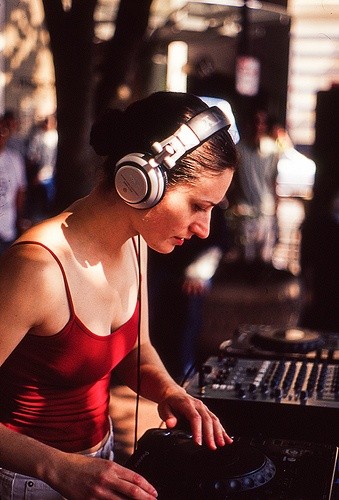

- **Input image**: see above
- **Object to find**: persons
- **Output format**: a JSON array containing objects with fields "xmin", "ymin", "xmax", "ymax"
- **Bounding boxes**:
[
  {"xmin": 0, "ymin": 91, "xmax": 236, "ymax": 498},
  {"xmin": 1, "ymin": 100, "xmax": 316, "ymax": 382}
]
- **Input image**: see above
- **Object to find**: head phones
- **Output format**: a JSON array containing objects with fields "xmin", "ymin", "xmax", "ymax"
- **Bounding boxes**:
[{"xmin": 113, "ymin": 106, "xmax": 232, "ymax": 209}]
